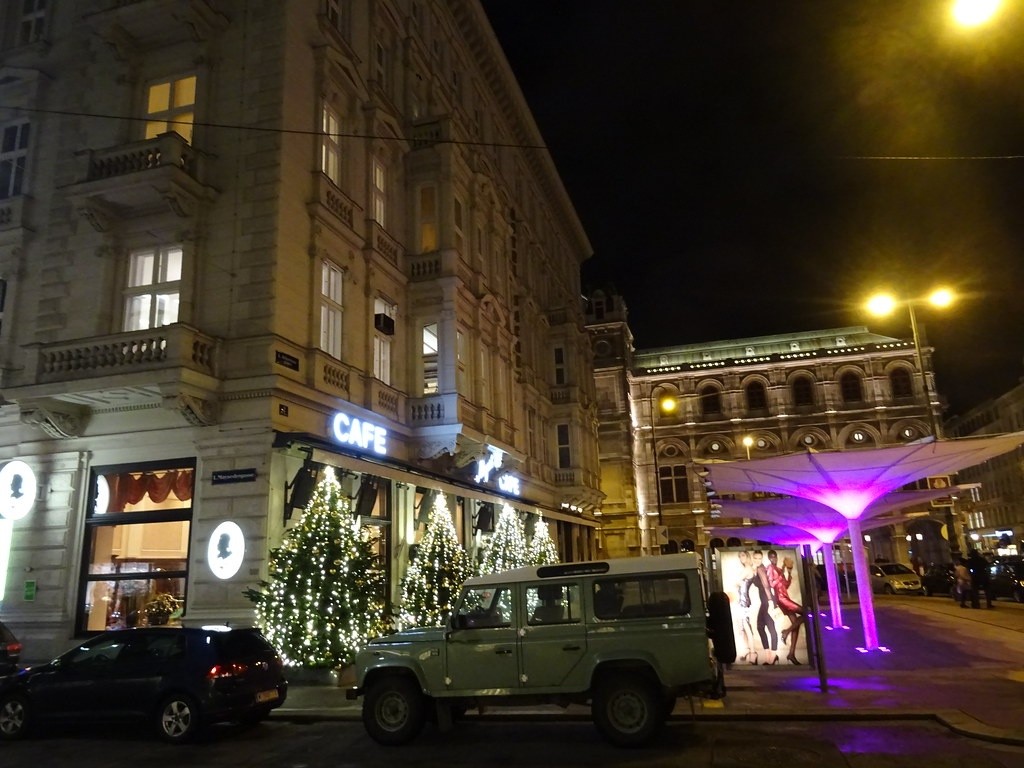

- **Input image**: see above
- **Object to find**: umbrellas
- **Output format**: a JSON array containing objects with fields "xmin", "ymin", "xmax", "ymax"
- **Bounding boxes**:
[{"xmin": 698, "ymin": 431, "xmax": 1024, "ymax": 647}]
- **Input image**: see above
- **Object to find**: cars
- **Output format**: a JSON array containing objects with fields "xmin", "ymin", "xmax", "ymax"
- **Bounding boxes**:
[
  {"xmin": 972, "ymin": 562, "xmax": 1024, "ymax": 603},
  {"xmin": 923, "ymin": 562, "xmax": 976, "ymax": 596},
  {"xmin": 870, "ymin": 563, "xmax": 926, "ymax": 594},
  {"xmin": 0, "ymin": 625, "xmax": 288, "ymax": 742},
  {"xmin": 836, "ymin": 563, "xmax": 856, "ymax": 588},
  {"xmin": 84, "ymin": 603, "xmax": 121, "ymax": 624},
  {"xmin": 125, "ymin": 600, "xmax": 184, "ymax": 626},
  {"xmin": 0, "ymin": 620, "xmax": 28, "ymax": 690}
]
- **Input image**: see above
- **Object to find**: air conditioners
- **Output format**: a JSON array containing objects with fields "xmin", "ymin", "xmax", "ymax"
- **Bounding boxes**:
[{"xmin": 374, "ymin": 313, "xmax": 395, "ymax": 336}]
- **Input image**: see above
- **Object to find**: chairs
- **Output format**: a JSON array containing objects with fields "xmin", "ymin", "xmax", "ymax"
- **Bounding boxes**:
[
  {"xmin": 594, "ymin": 583, "xmax": 624, "ymax": 620},
  {"xmin": 530, "ymin": 585, "xmax": 565, "ymax": 624}
]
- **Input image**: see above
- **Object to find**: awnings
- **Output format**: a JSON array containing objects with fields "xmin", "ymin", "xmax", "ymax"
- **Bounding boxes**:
[{"xmin": 301, "ymin": 438, "xmax": 603, "ymax": 527}]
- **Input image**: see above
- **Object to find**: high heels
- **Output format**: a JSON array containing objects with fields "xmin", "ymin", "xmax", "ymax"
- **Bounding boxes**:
[
  {"xmin": 786, "ymin": 655, "xmax": 802, "ymax": 665},
  {"xmin": 762, "ymin": 655, "xmax": 780, "ymax": 665},
  {"xmin": 781, "ymin": 630, "xmax": 787, "ymax": 645},
  {"xmin": 740, "ymin": 649, "xmax": 758, "ymax": 665}
]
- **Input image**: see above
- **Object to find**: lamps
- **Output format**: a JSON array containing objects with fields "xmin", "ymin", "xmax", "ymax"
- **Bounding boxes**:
[
  {"xmin": 414, "ymin": 488, "xmax": 438, "ymax": 529},
  {"xmin": 348, "ymin": 474, "xmax": 380, "ymax": 517},
  {"xmin": 284, "ymin": 461, "xmax": 320, "ymax": 527},
  {"xmin": 471, "ymin": 502, "xmax": 495, "ymax": 536}
]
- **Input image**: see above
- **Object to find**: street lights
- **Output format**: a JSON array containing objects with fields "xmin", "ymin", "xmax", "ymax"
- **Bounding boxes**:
[
  {"xmin": 869, "ymin": 289, "xmax": 964, "ymax": 564},
  {"xmin": 650, "ymin": 385, "xmax": 677, "ymax": 526},
  {"xmin": 742, "ymin": 435, "xmax": 754, "ymax": 461}
]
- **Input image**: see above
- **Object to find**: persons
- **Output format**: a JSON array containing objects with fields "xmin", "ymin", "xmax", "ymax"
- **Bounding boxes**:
[
  {"xmin": 737, "ymin": 549, "xmax": 807, "ymax": 666},
  {"xmin": 956, "ymin": 549, "xmax": 996, "ymax": 610}
]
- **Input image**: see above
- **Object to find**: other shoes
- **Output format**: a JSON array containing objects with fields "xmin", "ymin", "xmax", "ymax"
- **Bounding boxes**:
[{"xmin": 959, "ymin": 603, "xmax": 970, "ymax": 608}]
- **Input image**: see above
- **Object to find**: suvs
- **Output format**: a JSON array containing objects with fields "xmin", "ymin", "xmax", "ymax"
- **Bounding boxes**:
[{"xmin": 346, "ymin": 552, "xmax": 735, "ymax": 747}]
